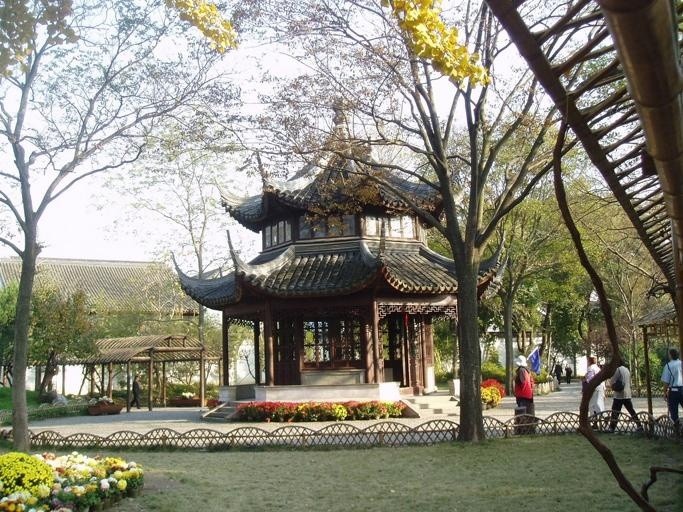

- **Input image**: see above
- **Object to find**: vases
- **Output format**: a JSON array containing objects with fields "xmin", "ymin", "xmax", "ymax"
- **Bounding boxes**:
[
  {"xmin": 86, "ymin": 403, "xmax": 124, "ymax": 415},
  {"xmin": 481, "ymin": 402, "xmax": 487, "ymax": 409}
]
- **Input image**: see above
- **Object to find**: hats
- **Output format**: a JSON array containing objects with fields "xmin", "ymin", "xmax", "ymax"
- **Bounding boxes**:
[{"xmin": 514, "ymin": 355, "xmax": 527, "ymax": 366}]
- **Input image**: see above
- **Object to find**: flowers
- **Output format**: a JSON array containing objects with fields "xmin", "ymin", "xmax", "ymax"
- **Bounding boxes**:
[
  {"xmin": 0, "ymin": 445, "xmax": 143, "ymax": 512},
  {"xmin": 479, "ymin": 378, "xmax": 505, "ymax": 406},
  {"xmin": 234, "ymin": 401, "xmax": 407, "ymax": 422},
  {"xmin": 87, "ymin": 396, "xmax": 113, "ymax": 406}
]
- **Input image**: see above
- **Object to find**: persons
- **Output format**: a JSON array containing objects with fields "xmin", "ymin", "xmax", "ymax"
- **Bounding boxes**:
[
  {"xmin": 565, "ymin": 364, "xmax": 572, "ymax": 384},
  {"xmin": 512, "ymin": 355, "xmax": 534, "ymax": 416},
  {"xmin": 582, "ymin": 356, "xmax": 606, "ymax": 429},
  {"xmin": 606, "ymin": 359, "xmax": 644, "ymax": 432},
  {"xmin": 661, "ymin": 349, "xmax": 683, "ymax": 424},
  {"xmin": 130, "ymin": 375, "xmax": 140, "ymax": 409},
  {"xmin": 554, "ymin": 364, "xmax": 563, "ymax": 384}
]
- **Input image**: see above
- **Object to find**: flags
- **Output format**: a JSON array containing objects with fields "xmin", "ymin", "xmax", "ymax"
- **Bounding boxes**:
[{"xmin": 529, "ymin": 346, "xmax": 541, "ymax": 374}]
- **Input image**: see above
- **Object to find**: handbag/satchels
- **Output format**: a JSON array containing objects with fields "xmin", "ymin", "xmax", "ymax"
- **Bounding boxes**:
[
  {"xmin": 582, "ymin": 381, "xmax": 590, "ymax": 390},
  {"xmin": 612, "ymin": 381, "xmax": 624, "ymax": 391}
]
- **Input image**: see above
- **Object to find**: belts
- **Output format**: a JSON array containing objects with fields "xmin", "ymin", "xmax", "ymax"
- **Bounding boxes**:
[{"xmin": 667, "ymin": 387, "xmax": 679, "ymax": 391}]
